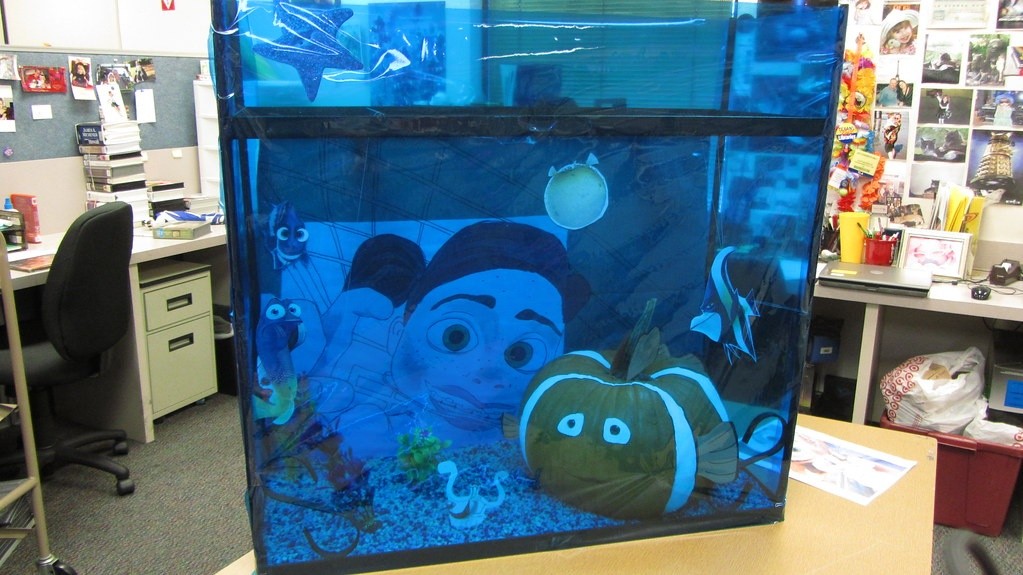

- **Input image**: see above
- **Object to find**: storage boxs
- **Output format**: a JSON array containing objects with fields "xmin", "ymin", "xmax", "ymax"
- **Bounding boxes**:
[
  {"xmin": 881, "ymin": 408, "xmax": 1023, "ymax": 535},
  {"xmin": 806, "ymin": 332, "xmax": 843, "ymax": 364}
]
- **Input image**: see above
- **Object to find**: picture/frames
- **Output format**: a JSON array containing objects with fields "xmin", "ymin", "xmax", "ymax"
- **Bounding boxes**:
[{"xmin": 900, "ymin": 228, "xmax": 973, "ymax": 280}]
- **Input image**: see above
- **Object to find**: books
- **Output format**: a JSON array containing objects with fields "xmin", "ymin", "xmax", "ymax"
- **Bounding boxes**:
[
  {"xmin": 10, "ymin": 194, "xmax": 41, "ymax": 244},
  {"xmin": 76, "ymin": 120, "xmax": 218, "ymax": 228},
  {"xmin": 153, "ymin": 221, "xmax": 212, "ymax": 240}
]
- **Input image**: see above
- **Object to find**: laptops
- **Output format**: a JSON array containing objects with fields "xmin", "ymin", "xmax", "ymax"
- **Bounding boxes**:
[{"xmin": 817, "ymin": 261, "xmax": 934, "ymax": 299}]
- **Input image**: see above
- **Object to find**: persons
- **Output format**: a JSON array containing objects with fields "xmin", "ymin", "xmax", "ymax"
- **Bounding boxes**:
[
  {"xmin": 123, "ymin": 66, "xmax": 131, "ymax": 81},
  {"xmin": 881, "ymin": 8, "xmax": 919, "ymax": 54},
  {"xmin": 884, "ymin": 113, "xmax": 902, "ymax": 159},
  {"xmin": 854, "ymin": 0, "xmax": 873, "ymax": 24},
  {"xmin": 936, "ymin": 95, "xmax": 951, "ymax": 123},
  {"xmin": 74, "ymin": 63, "xmax": 89, "ymax": 82},
  {"xmin": 27, "ymin": 69, "xmax": 50, "ymax": 89},
  {"xmin": 929, "ymin": 53, "xmax": 952, "ymax": 70},
  {"xmin": 0, "ymin": 98, "xmax": 6, "ymax": 117},
  {"xmin": 102, "ymin": 69, "xmax": 120, "ymax": 83},
  {"xmin": 883, "ymin": 185, "xmax": 900, "ymax": 197},
  {"xmin": 879, "ymin": 78, "xmax": 912, "ymax": 107},
  {"xmin": 995, "ymin": 52, "xmax": 1005, "ymax": 81},
  {"xmin": 993, "ymin": 98, "xmax": 1012, "ymax": 125},
  {"xmin": 134, "ymin": 63, "xmax": 148, "ymax": 82},
  {"xmin": 106, "ymin": 86, "xmax": 118, "ymax": 101}
]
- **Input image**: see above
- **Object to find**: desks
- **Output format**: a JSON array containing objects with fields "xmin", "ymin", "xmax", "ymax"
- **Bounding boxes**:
[
  {"xmin": 816, "ymin": 260, "xmax": 1023, "ymax": 427},
  {"xmin": 213, "ymin": 404, "xmax": 939, "ymax": 575},
  {"xmin": 0, "ymin": 225, "xmax": 227, "ymax": 444}
]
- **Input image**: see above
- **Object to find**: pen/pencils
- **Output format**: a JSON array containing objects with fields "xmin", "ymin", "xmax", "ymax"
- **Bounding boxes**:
[
  {"xmin": 857, "ymin": 222, "xmax": 869, "ymax": 237},
  {"xmin": 878, "ymin": 217, "xmax": 900, "ymax": 241},
  {"xmin": 822, "ymin": 223, "xmax": 840, "ymax": 252}
]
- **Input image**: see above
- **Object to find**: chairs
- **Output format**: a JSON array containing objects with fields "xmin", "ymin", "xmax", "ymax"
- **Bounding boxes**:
[{"xmin": 0, "ymin": 202, "xmax": 136, "ymax": 495}]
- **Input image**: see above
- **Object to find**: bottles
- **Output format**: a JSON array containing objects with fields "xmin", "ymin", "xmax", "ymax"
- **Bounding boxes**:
[{"xmin": 3, "ymin": 198, "xmax": 23, "ymax": 244}]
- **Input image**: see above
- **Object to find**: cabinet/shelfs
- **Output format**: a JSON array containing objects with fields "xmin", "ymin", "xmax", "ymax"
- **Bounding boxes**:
[
  {"xmin": 139, "ymin": 260, "xmax": 220, "ymax": 421},
  {"xmin": 0, "ymin": 230, "xmax": 78, "ymax": 575},
  {"xmin": 193, "ymin": 80, "xmax": 220, "ymax": 198}
]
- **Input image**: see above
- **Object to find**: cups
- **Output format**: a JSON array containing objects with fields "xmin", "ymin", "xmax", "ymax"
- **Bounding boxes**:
[
  {"xmin": 865, "ymin": 235, "xmax": 897, "ymax": 266},
  {"xmin": 838, "ymin": 211, "xmax": 872, "ymax": 264}
]
